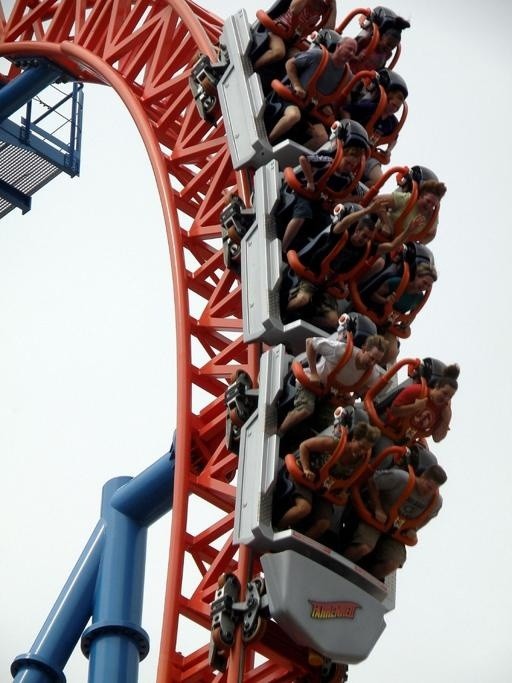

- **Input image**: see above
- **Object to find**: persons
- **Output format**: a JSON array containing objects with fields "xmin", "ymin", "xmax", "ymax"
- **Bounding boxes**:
[{"xmin": 252, "ymin": 1, "xmax": 461, "ymax": 584}]
